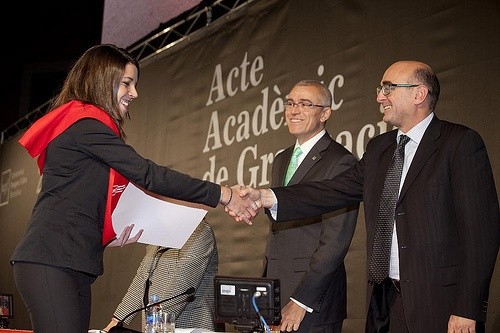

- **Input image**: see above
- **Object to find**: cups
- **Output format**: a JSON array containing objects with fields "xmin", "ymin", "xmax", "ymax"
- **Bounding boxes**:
[{"xmin": 156, "ymin": 312, "xmax": 176, "ymax": 333}]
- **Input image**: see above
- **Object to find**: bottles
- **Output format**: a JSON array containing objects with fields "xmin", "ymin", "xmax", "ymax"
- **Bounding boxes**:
[{"xmin": 145, "ymin": 295, "xmax": 162, "ymax": 333}]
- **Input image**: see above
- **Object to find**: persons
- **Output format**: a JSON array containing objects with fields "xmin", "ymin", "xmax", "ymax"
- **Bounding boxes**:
[
  {"xmin": 102, "ymin": 216, "xmax": 218, "ymax": 333},
  {"xmin": 260, "ymin": 79, "xmax": 362, "ymax": 333},
  {"xmin": 12, "ymin": 44, "xmax": 256, "ymax": 333},
  {"xmin": 224, "ymin": 60, "xmax": 500, "ymax": 333}
]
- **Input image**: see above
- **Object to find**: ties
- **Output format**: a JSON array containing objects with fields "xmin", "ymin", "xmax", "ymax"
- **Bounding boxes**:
[
  {"xmin": 284, "ymin": 147, "xmax": 302, "ymax": 187},
  {"xmin": 368, "ymin": 134, "xmax": 411, "ymax": 285}
]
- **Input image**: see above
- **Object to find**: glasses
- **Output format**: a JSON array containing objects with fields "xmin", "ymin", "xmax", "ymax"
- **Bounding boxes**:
[
  {"xmin": 377, "ymin": 82, "xmax": 431, "ymax": 95},
  {"xmin": 283, "ymin": 99, "xmax": 324, "ymax": 110}
]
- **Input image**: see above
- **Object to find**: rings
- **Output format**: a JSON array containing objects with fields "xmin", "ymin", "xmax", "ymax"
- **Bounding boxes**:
[{"xmin": 251, "ymin": 203, "xmax": 257, "ymax": 209}]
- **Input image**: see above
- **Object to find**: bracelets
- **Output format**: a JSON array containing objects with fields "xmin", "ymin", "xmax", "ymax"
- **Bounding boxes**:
[{"xmin": 221, "ymin": 187, "xmax": 233, "ymax": 206}]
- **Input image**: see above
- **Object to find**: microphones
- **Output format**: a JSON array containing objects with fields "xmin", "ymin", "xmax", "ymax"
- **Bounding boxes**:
[{"xmin": 107, "ymin": 287, "xmax": 196, "ymax": 333}]
